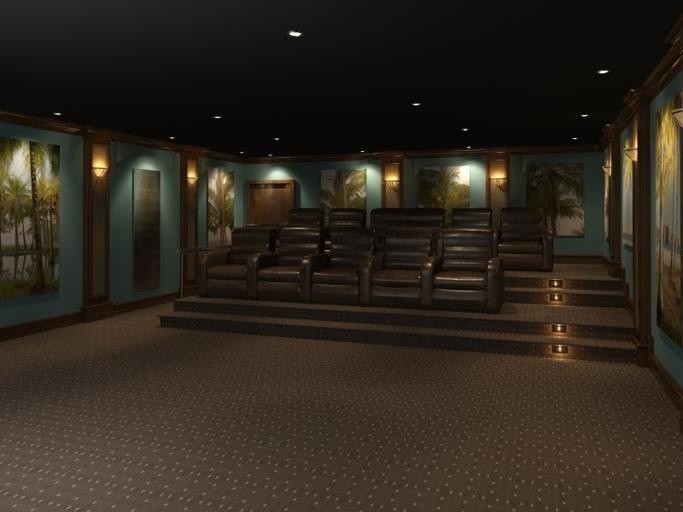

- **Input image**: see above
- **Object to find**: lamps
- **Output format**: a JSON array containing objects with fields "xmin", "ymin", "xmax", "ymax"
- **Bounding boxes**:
[
  {"xmin": 623, "ymin": 148, "xmax": 637, "ymax": 163},
  {"xmin": 92, "ymin": 166, "xmax": 107, "ymax": 178},
  {"xmin": 186, "ymin": 176, "xmax": 198, "ymax": 185},
  {"xmin": 670, "ymin": 107, "xmax": 683, "ymax": 128},
  {"xmin": 602, "ymin": 166, "xmax": 611, "ymax": 176}
]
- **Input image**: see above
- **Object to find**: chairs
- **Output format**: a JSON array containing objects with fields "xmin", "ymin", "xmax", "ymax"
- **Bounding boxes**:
[
  {"xmin": 194, "ymin": 204, "xmax": 501, "ymax": 314},
  {"xmin": 496, "ymin": 207, "xmax": 555, "ymax": 273}
]
